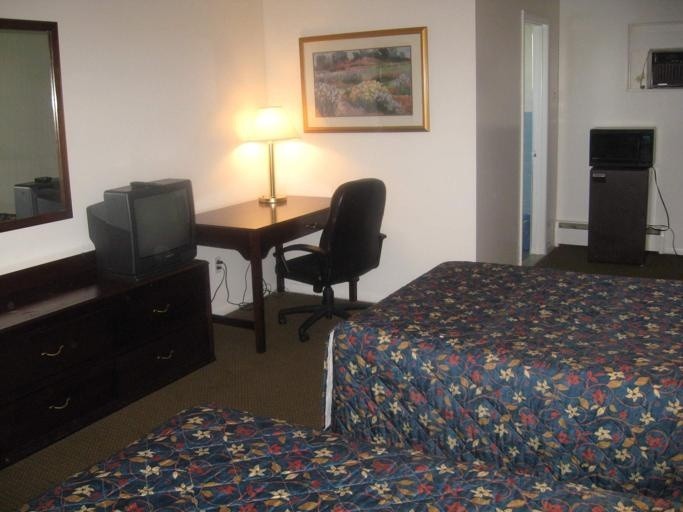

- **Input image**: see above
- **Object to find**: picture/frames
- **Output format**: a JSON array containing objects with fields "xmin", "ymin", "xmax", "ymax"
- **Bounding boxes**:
[{"xmin": 298, "ymin": 26, "xmax": 430, "ymax": 132}]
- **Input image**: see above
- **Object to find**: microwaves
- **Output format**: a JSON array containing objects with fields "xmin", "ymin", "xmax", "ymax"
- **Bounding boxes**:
[{"xmin": 590, "ymin": 129, "xmax": 653, "ymax": 169}]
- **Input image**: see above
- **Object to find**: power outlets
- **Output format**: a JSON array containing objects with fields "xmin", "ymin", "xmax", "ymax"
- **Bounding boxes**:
[{"xmin": 215, "ymin": 256, "xmax": 222, "ymax": 273}]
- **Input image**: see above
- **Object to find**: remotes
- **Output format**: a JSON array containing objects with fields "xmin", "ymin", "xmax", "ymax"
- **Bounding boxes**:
[
  {"xmin": 35, "ymin": 177, "xmax": 53, "ymax": 182},
  {"xmin": 130, "ymin": 181, "xmax": 163, "ymax": 188}
]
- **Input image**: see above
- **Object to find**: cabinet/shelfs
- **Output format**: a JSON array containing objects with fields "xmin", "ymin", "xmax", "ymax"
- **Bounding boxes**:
[
  {"xmin": 588, "ymin": 167, "xmax": 649, "ymax": 265},
  {"xmin": 0, "ymin": 259, "xmax": 216, "ymax": 470}
]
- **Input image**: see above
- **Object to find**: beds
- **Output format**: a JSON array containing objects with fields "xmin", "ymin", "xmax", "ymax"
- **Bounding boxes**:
[
  {"xmin": 18, "ymin": 402, "xmax": 683, "ymax": 512},
  {"xmin": 322, "ymin": 261, "xmax": 683, "ymax": 503}
]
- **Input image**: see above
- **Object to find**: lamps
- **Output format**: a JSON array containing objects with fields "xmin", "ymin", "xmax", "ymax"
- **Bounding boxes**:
[
  {"xmin": 259, "ymin": 202, "xmax": 288, "ymax": 224},
  {"xmin": 245, "ymin": 105, "xmax": 296, "ymax": 204}
]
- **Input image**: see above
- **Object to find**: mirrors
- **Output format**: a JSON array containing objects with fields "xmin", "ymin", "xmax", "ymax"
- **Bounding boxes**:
[{"xmin": 0, "ymin": 17, "xmax": 72, "ymax": 233}]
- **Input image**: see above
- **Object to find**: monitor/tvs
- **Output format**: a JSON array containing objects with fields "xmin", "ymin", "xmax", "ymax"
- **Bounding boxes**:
[
  {"xmin": 14, "ymin": 178, "xmax": 61, "ymax": 220},
  {"xmin": 86, "ymin": 179, "xmax": 197, "ymax": 283}
]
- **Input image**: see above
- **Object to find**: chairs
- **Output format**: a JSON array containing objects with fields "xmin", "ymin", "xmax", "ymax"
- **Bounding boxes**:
[{"xmin": 273, "ymin": 179, "xmax": 387, "ymax": 342}]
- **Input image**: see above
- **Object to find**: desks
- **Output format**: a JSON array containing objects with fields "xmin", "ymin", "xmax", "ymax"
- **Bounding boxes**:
[{"xmin": 193, "ymin": 196, "xmax": 333, "ymax": 353}]
